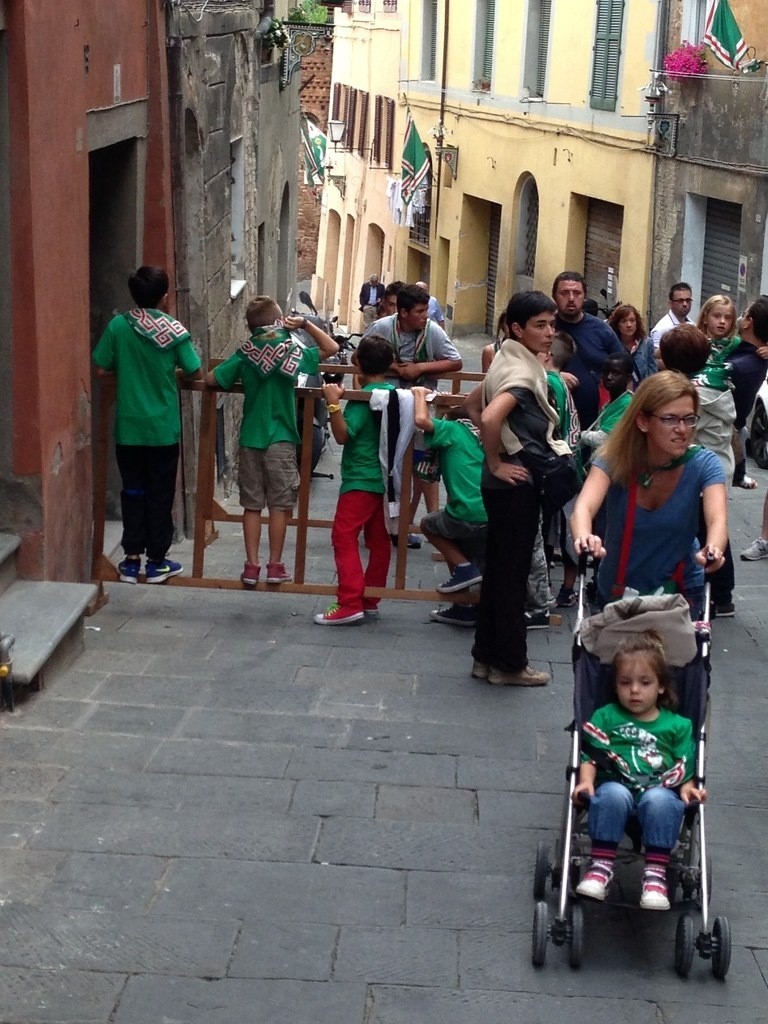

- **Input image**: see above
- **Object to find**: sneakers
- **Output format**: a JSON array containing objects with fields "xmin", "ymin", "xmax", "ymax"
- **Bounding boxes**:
[
  {"xmin": 711, "ymin": 602, "xmax": 736, "ymax": 616},
  {"xmin": 524, "ymin": 609, "xmax": 551, "ymax": 630},
  {"xmin": 556, "ymin": 583, "xmax": 577, "ymax": 608},
  {"xmin": 575, "ymin": 863, "xmax": 614, "ymax": 901},
  {"xmin": 239, "ymin": 559, "xmax": 262, "ymax": 586},
  {"xmin": 116, "ymin": 557, "xmax": 142, "ymax": 584},
  {"xmin": 437, "ymin": 562, "xmax": 484, "ymax": 595},
  {"xmin": 362, "ymin": 606, "xmax": 379, "ymax": 615},
  {"xmin": 264, "ymin": 561, "xmax": 294, "ymax": 584},
  {"xmin": 471, "ymin": 658, "xmax": 491, "ymax": 680},
  {"xmin": 639, "ymin": 870, "xmax": 672, "ymax": 911},
  {"xmin": 406, "ymin": 532, "xmax": 422, "ymax": 549},
  {"xmin": 313, "ymin": 600, "xmax": 365, "ymax": 625},
  {"xmin": 144, "ymin": 558, "xmax": 184, "ymax": 584},
  {"xmin": 740, "ymin": 536, "xmax": 768, "ymax": 562},
  {"xmin": 486, "ymin": 662, "xmax": 551, "ymax": 687},
  {"xmin": 430, "ymin": 602, "xmax": 476, "ymax": 626}
]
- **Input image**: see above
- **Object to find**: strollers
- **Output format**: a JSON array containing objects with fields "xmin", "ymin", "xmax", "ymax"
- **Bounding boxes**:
[{"xmin": 531, "ymin": 549, "xmax": 731, "ymax": 979}]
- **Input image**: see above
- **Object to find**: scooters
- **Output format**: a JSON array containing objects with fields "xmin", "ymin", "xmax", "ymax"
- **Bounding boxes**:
[
  {"xmin": 290, "ymin": 291, "xmax": 363, "ymax": 473},
  {"xmin": 598, "ymin": 288, "xmax": 623, "ymax": 319}
]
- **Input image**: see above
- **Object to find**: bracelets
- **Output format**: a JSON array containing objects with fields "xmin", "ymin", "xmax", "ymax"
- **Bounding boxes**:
[{"xmin": 301, "ymin": 318, "xmax": 307, "ymax": 328}]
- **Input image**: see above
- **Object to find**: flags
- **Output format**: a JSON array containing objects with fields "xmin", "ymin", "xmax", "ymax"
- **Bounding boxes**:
[
  {"xmin": 300, "ymin": 120, "xmax": 326, "ymax": 198},
  {"xmin": 704, "ymin": 0, "xmax": 760, "ymax": 73},
  {"xmin": 401, "ymin": 109, "xmax": 431, "ymax": 206}
]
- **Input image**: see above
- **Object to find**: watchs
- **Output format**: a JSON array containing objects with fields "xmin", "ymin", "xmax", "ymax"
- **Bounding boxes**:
[{"xmin": 326, "ymin": 404, "xmax": 339, "ymax": 413}]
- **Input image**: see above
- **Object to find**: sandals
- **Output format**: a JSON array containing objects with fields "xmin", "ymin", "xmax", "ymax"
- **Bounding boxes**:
[{"xmin": 733, "ymin": 476, "xmax": 759, "ymax": 490}]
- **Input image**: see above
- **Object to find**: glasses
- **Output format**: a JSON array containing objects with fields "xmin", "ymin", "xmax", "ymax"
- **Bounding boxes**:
[
  {"xmin": 669, "ymin": 298, "xmax": 693, "ymax": 304},
  {"xmin": 648, "ymin": 412, "xmax": 701, "ymax": 428}
]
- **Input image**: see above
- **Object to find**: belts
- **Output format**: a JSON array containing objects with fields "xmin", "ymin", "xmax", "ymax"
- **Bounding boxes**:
[{"xmin": 366, "ymin": 303, "xmax": 377, "ymax": 307}]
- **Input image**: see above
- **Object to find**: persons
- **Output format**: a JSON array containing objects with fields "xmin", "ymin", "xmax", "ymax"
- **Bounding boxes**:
[
  {"xmin": 411, "ymin": 387, "xmax": 490, "ymax": 626},
  {"xmin": 581, "ymin": 351, "xmax": 635, "ymax": 449},
  {"xmin": 314, "ymin": 335, "xmax": 396, "ymax": 625},
  {"xmin": 482, "ymin": 309, "xmax": 510, "ymax": 373},
  {"xmin": 552, "ymin": 271, "xmax": 624, "ymax": 431},
  {"xmin": 359, "ymin": 274, "xmax": 385, "ymax": 329},
  {"xmin": 740, "ymin": 490, "xmax": 768, "ymax": 561},
  {"xmin": 568, "ymin": 370, "xmax": 729, "ymax": 621},
  {"xmin": 571, "ymin": 630, "xmax": 708, "ymax": 911},
  {"xmin": 92, "ymin": 265, "xmax": 203, "ymax": 585},
  {"xmin": 659, "ymin": 325, "xmax": 736, "ymax": 616},
  {"xmin": 650, "ymin": 282, "xmax": 696, "ymax": 348},
  {"xmin": 607, "ymin": 304, "xmax": 658, "ymax": 378},
  {"xmin": 696, "ymin": 295, "xmax": 768, "ymax": 489},
  {"xmin": 525, "ymin": 332, "xmax": 584, "ymax": 628},
  {"xmin": 206, "ymin": 295, "xmax": 339, "ymax": 585},
  {"xmin": 351, "ymin": 281, "xmax": 463, "ymax": 549},
  {"xmin": 461, "ymin": 291, "xmax": 573, "ymax": 685}
]
